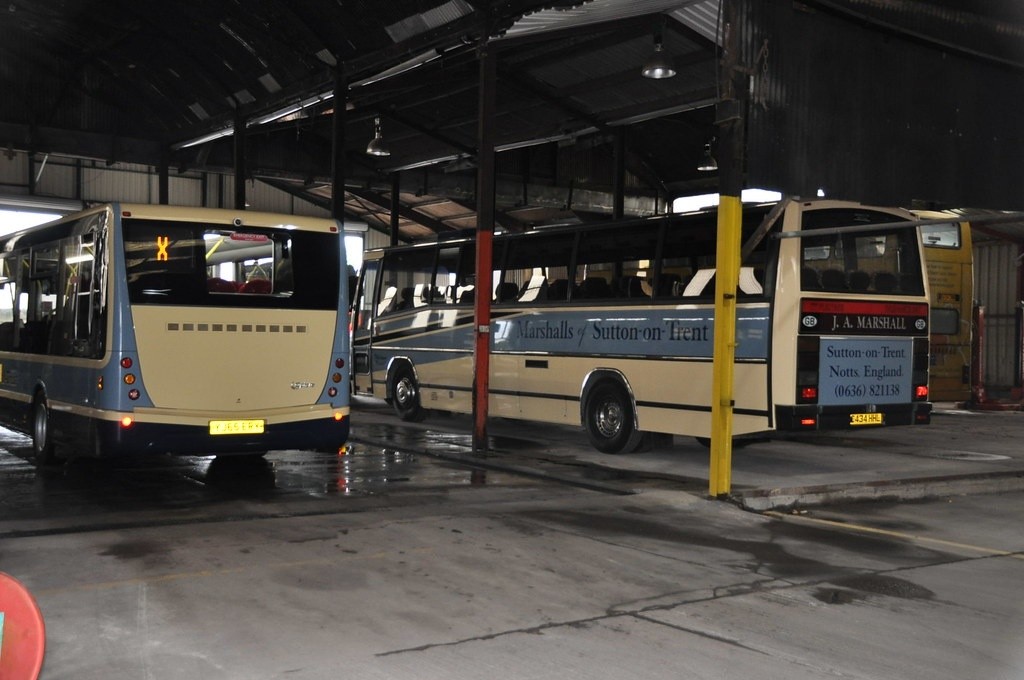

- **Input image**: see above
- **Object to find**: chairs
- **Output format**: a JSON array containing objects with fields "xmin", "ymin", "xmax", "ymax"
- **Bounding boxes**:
[
  {"xmin": 395, "ymin": 267, "xmax": 920, "ymax": 313},
  {"xmin": 207, "ymin": 275, "xmax": 273, "ymax": 294},
  {"xmin": 0, "ymin": 319, "xmax": 96, "ymax": 351}
]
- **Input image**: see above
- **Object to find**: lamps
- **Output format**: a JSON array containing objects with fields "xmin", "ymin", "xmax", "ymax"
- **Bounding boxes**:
[
  {"xmin": 366, "ymin": 116, "xmax": 390, "ymax": 156},
  {"xmin": 641, "ymin": 31, "xmax": 676, "ymax": 79},
  {"xmin": 696, "ymin": 140, "xmax": 718, "ymax": 171}
]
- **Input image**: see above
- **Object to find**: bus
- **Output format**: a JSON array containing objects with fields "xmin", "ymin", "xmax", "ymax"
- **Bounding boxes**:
[
  {"xmin": 801, "ymin": 206, "xmax": 977, "ymax": 414},
  {"xmin": 347, "ymin": 196, "xmax": 935, "ymax": 457},
  {"xmin": 0, "ymin": 202, "xmax": 354, "ymax": 482}
]
